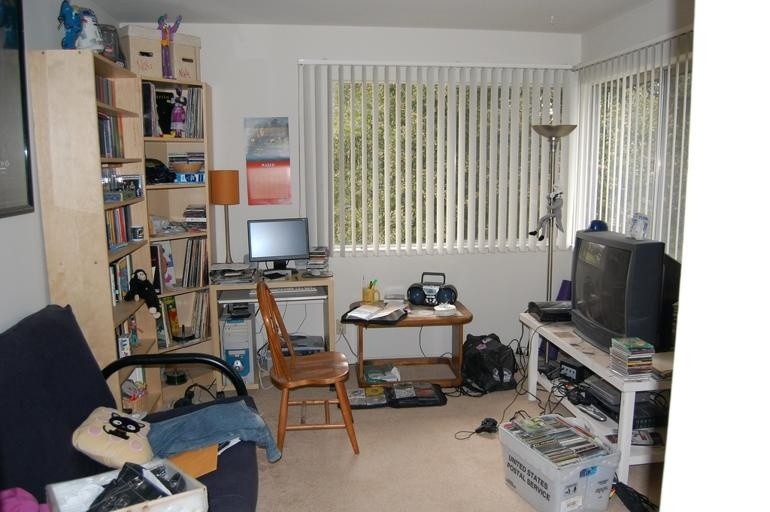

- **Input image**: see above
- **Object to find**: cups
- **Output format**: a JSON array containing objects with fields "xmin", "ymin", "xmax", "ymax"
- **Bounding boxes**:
[{"xmin": 362, "ymin": 287, "xmax": 381, "ymax": 306}]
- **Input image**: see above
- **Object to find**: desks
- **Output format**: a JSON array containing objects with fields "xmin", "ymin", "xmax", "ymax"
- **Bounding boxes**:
[
  {"xmin": 520, "ymin": 312, "xmax": 675, "ymax": 485},
  {"xmin": 207, "ymin": 269, "xmax": 336, "ymax": 391},
  {"xmin": 351, "ymin": 299, "xmax": 473, "ymax": 387}
]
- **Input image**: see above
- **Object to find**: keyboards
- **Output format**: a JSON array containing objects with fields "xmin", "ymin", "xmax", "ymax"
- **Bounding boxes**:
[{"xmin": 248, "ymin": 286, "xmax": 316, "ymax": 293}]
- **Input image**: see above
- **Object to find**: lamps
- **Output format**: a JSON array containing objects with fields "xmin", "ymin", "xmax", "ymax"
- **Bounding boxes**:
[
  {"xmin": 532, "ymin": 125, "xmax": 577, "ymax": 370},
  {"xmin": 209, "ymin": 169, "xmax": 242, "ymax": 278}
]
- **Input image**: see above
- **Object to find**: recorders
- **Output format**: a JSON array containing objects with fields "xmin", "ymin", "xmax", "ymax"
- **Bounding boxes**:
[{"xmin": 407, "ymin": 272, "xmax": 457, "ymax": 306}]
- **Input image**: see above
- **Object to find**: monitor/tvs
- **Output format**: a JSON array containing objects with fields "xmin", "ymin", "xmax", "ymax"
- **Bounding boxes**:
[
  {"xmin": 569, "ymin": 228, "xmax": 681, "ymax": 355},
  {"xmin": 247, "ymin": 217, "xmax": 309, "ymax": 276}
]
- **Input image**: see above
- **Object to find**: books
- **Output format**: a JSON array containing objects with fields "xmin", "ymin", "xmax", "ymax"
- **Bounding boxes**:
[
  {"xmin": 341, "ymin": 304, "xmax": 407, "ymax": 325},
  {"xmin": 94, "ymin": 75, "xmax": 209, "ymax": 359},
  {"xmin": 305, "ymin": 244, "xmax": 331, "ymax": 271}
]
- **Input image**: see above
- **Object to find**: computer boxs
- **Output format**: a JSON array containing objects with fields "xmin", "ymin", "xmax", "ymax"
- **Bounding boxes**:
[{"xmin": 219, "ymin": 304, "xmax": 257, "ymax": 385}]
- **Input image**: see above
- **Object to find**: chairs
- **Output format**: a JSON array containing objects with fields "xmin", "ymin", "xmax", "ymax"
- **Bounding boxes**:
[
  {"xmin": 0, "ymin": 305, "xmax": 258, "ymax": 512},
  {"xmin": 257, "ymin": 281, "xmax": 359, "ymax": 456}
]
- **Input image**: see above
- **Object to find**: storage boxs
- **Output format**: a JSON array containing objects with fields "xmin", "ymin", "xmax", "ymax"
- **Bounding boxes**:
[
  {"xmin": 501, "ymin": 416, "xmax": 621, "ymax": 512},
  {"xmin": 43, "ymin": 458, "xmax": 209, "ymax": 512},
  {"xmin": 119, "ymin": 24, "xmax": 201, "ymax": 82}
]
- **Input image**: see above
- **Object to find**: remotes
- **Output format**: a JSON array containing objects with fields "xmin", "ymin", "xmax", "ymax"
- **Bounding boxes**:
[{"xmin": 580, "ymin": 404, "xmax": 607, "ymax": 422}]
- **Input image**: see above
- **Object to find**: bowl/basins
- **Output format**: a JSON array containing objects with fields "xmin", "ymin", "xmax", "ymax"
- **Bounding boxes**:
[{"xmin": 170, "ymin": 162, "xmax": 202, "ymax": 173}]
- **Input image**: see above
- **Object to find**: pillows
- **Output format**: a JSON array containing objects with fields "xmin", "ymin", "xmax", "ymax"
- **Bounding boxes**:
[{"xmin": 72, "ymin": 406, "xmax": 160, "ymax": 467}]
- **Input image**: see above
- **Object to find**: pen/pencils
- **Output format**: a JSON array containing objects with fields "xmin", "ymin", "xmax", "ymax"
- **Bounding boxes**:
[
  {"xmin": 182, "ymin": 324, "xmax": 185, "ymax": 340},
  {"xmin": 368, "ymin": 280, "xmax": 378, "ymax": 289}
]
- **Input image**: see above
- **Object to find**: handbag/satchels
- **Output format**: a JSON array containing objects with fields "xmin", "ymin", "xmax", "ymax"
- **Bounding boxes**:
[
  {"xmin": 85, "ymin": 462, "xmax": 185, "ymax": 512},
  {"xmin": 460, "ymin": 333, "xmax": 517, "ymax": 395}
]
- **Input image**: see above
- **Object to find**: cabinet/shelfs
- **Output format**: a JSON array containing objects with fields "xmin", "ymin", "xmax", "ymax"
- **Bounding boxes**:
[{"xmin": 30, "ymin": 49, "xmax": 216, "ymax": 418}]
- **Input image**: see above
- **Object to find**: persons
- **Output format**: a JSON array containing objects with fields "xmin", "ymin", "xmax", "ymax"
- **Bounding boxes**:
[{"xmin": 158, "ymin": 12, "xmax": 183, "ymax": 80}]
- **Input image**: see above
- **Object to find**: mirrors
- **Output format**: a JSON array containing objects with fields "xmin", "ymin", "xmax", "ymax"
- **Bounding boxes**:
[{"xmin": 0, "ymin": 0, "xmax": 35, "ymax": 217}]
